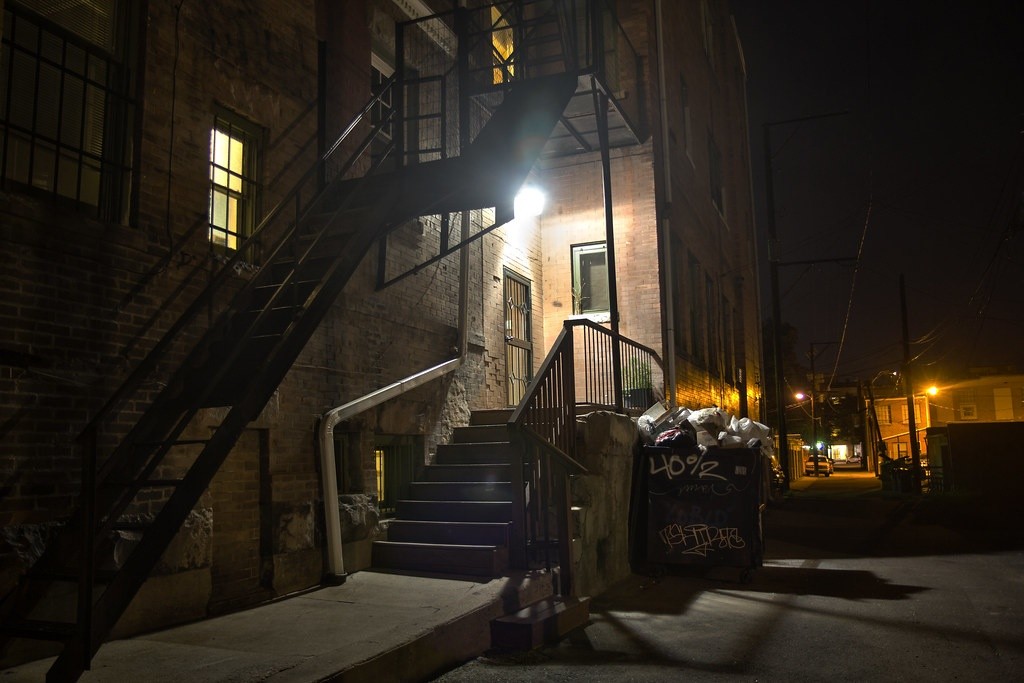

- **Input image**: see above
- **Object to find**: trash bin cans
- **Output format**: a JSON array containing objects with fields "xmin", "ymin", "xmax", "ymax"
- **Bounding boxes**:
[{"xmin": 642, "ymin": 442, "xmax": 769, "ymax": 572}]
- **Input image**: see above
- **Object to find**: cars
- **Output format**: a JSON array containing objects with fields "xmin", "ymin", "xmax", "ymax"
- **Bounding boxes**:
[
  {"xmin": 846, "ymin": 455, "xmax": 861, "ymax": 464},
  {"xmin": 805, "ymin": 455, "xmax": 834, "ymax": 477}
]
